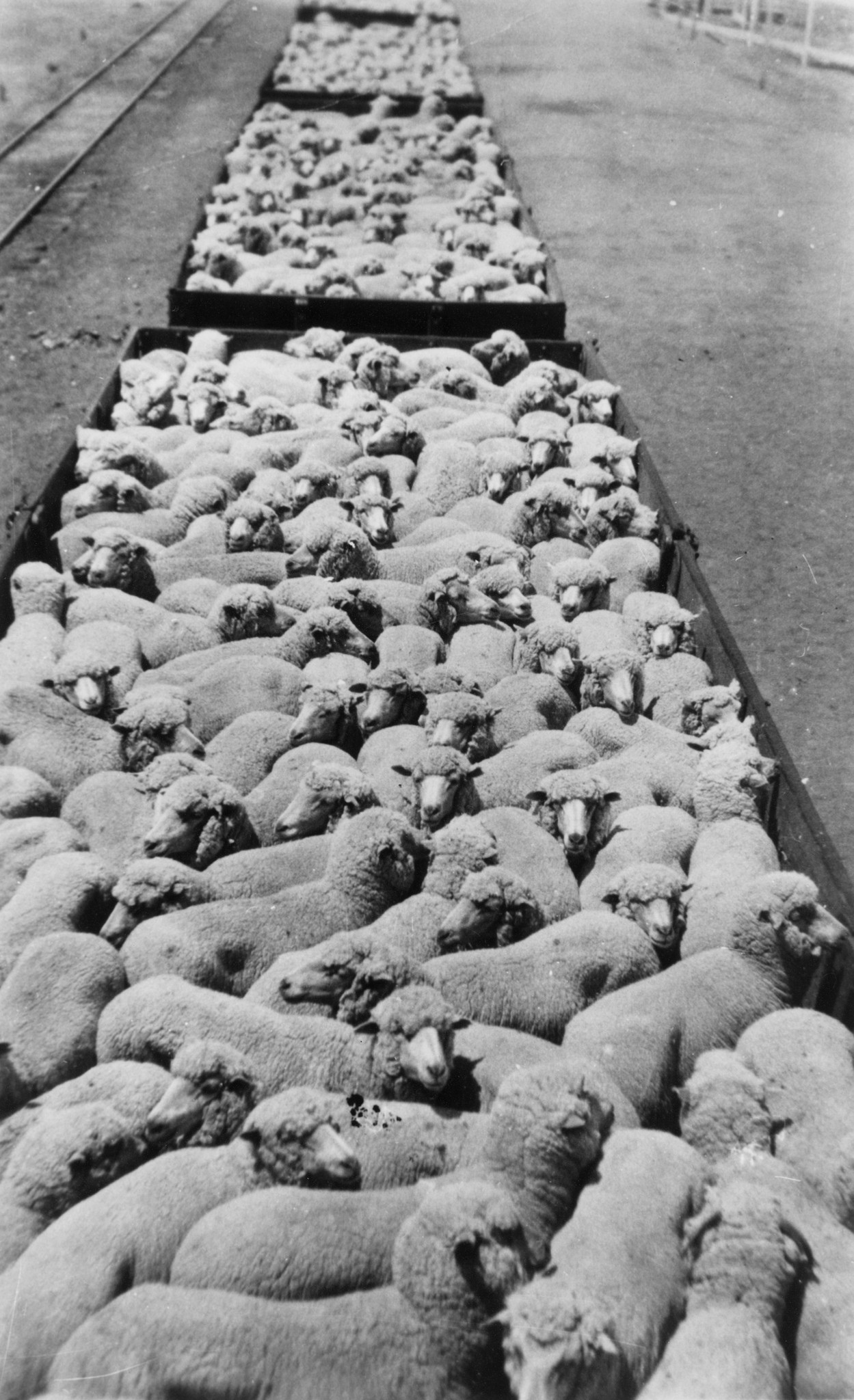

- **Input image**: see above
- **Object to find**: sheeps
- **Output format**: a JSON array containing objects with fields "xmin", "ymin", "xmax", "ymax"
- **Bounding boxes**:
[
  {"xmin": 184, "ymin": 93, "xmax": 551, "ymax": 303},
  {"xmin": 0, "ymin": 327, "xmax": 853, "ymax": 1400},
  {"xmin": 297, "ymin": 0, "xmax": 458, "ymax": 19},
  {"xmin": 272, "ymin": 10, "xmax": 476, "ymax": 98}
]
